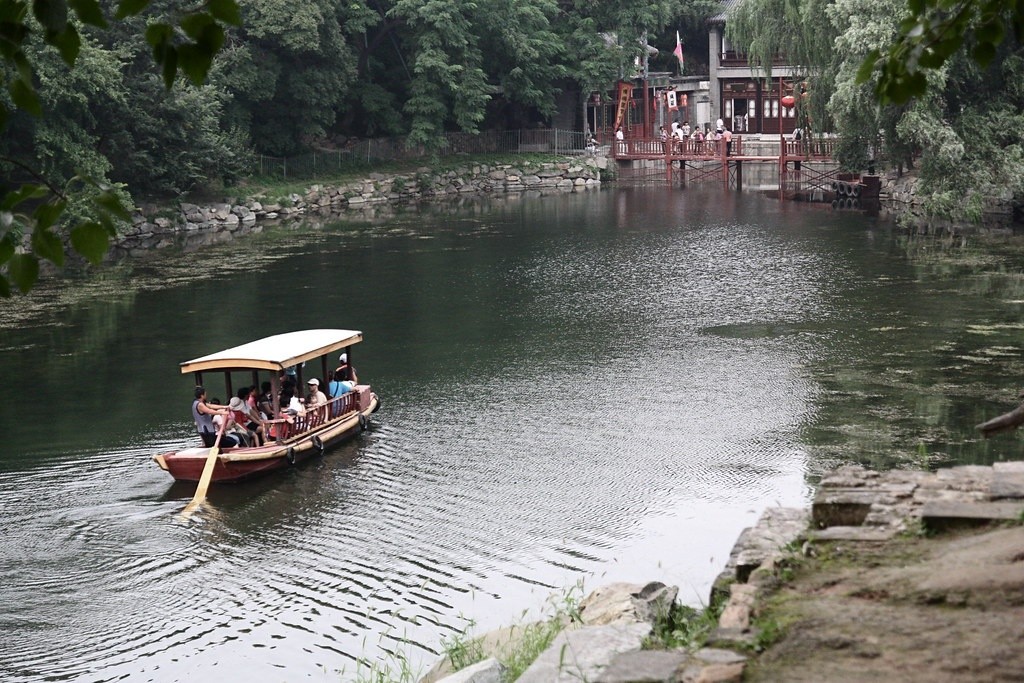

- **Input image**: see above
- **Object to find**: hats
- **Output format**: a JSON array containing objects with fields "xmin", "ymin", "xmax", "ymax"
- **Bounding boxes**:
[
  {"xmin": 286, "ymin": 368, "xmax": 296, "ymax": 376},
  {"xmin": 339, "ymin": 353, "xmax": 347, "ymax": 363},
  {"xmin": 229, "ymin": 397, "xmax": 244, "ymax": 411},
  {"xmin": 307, "ymin": 378, "xmax": 320, "ymax": 386}
]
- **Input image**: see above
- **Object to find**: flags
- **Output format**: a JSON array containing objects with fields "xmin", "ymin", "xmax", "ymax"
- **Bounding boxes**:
[{"xmin": 674, "ymin": 43, "xmax": 684, "ymax": 64}]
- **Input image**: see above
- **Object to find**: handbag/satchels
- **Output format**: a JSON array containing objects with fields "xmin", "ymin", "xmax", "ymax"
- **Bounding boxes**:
[{"xmin": 233, "ymin": 420, "xmax": 247, "ymax": 434}]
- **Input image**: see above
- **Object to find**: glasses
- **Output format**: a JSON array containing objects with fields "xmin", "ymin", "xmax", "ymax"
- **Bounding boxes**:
[{"xmin": 308, "ymin": 383, "xmax": 316, "ymax": 386}]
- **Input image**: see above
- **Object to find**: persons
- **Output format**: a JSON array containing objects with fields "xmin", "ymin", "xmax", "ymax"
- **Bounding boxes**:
[
  {"xmin": 192, "ymin": 386, "xmax": 232, "ymax": 447},
  {"xmin": 208, "ymin": 352, "xmax": 359, "ymax": 448},
  {"xmin": 585, "ymin": 113, "xmax": 803, "ymax": 156}
]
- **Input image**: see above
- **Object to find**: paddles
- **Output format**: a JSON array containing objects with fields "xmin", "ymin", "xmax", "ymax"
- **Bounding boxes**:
[{"xmin": 180, "ymin": 406, "xmax": 230, "ymax": 520}]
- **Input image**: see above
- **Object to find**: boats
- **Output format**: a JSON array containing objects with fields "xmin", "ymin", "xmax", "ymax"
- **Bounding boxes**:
[{"xmin": 151, "ymin": 328, "xmax": 382, "ymax": 485}]
